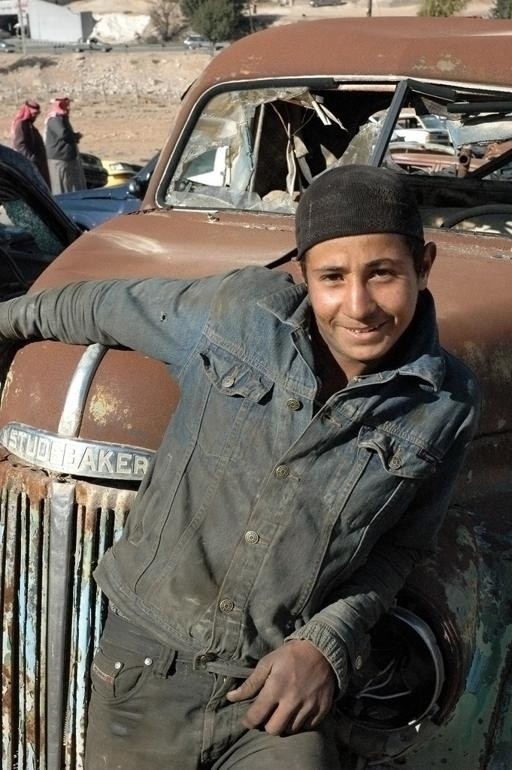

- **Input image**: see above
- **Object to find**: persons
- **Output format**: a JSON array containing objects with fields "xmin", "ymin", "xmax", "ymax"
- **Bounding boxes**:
[
  {"xmin": 11, "ymin": 100, "xmax": 46, "ymax": 176},
  {"xmin": 1, "ymin": 164, "xmax": 479, "ymax": 768},
  {"xmin": 44, "ymin": 94, "xmax": 89, "ymax": 197}
]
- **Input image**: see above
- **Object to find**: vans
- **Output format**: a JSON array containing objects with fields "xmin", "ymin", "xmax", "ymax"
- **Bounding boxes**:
[{"xmin": 0, "ymin": 16, "xmax": 512, "ymax": 769}]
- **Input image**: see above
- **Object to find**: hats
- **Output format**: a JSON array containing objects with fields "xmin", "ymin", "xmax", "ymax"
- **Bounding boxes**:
[{"xmin": 293, "ymin": 163, "xmax": 425, "ymax": 261}]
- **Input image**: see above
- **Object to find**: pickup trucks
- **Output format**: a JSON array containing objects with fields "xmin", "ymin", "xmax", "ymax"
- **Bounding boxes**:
[{"xmin": 70, "ymin": 37, "xmax": 113, "ymax": 54}]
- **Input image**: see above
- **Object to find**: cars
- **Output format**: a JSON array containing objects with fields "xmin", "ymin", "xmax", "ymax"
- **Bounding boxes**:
[
  {"xmin": 1, "ymin": 28, "xmax": 11, "ymax": 39},
  {"xmin": 215, "ymin": 41, "xmax": 231, "ymax": 51},
  {"xmin": 1, "ymin": 40, "xmax": 16, "ymax": 52}
]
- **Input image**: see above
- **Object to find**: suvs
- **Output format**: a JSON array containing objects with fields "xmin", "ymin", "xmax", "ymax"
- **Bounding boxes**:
[
  {"xmin": 311, "ymin": 0, "xmax": 342, "ymax": 7},
  {"xmin": 185, "ymin": 35, "xmax": 214, "ymax": 49}
]
